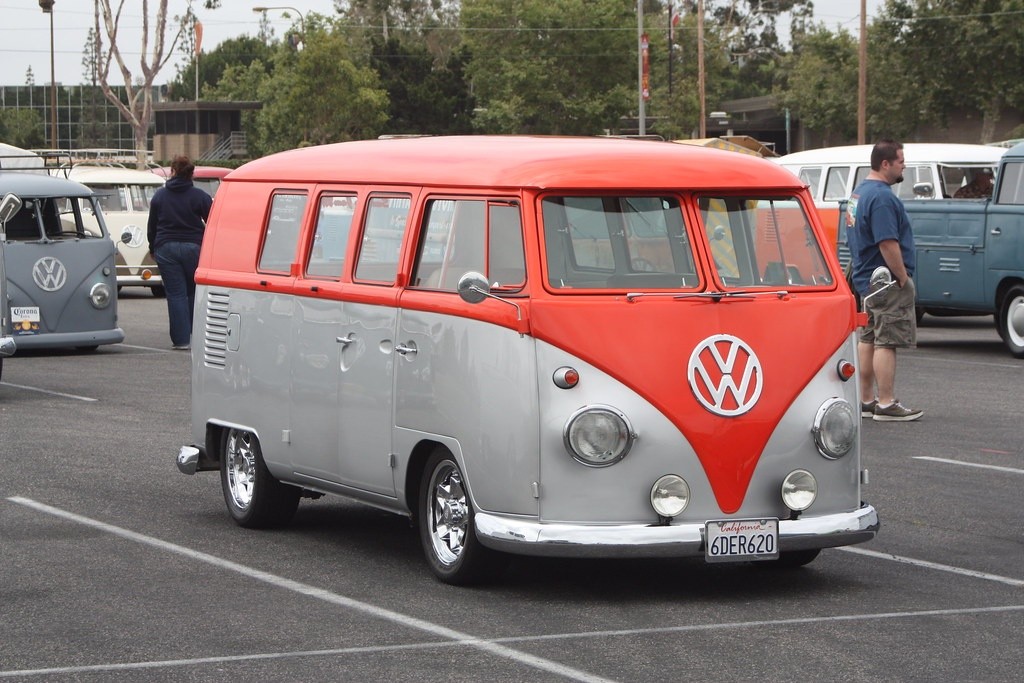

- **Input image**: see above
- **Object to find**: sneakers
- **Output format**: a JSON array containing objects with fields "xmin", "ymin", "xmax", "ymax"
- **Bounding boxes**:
[
  {"xmin": 861, "ymin": 398, "xmax": 879, "ymax": 418},
  {"xmin": 872, "ymin": 399, "xmax": 924, "ymax": 421}
]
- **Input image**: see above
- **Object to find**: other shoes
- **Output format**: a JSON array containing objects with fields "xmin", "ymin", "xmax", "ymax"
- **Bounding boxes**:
[{"xmin": 171, "ymin": 342, "xmax": 188, "ymax": 350}]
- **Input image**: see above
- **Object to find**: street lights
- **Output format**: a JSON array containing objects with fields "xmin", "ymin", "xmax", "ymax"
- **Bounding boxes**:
[
  {"xmin": 252, "ymin": 5, "xmax": 306, "ymax": 51},
  {"xmin": 37, "ymin": 0, "xmax": 58, "ymax": 151}
]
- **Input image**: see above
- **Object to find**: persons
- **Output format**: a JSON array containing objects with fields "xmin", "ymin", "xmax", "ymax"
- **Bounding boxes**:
[
  {"xmin": 954, "ymin": 168, "xmax": 995, "ymax": 198},
  {"xmin": 147, "ymin": 155, "xmax": 212, "ymax": 349},
  {"xmin": 846, "ymin": 138, "xmax": 924, "ymax": 421}
]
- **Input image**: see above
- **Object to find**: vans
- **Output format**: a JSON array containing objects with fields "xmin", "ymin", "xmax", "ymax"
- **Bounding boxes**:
[
  {"xmin": 46, "ymin": 162, "xmax": 169, "ymax": 300},
  {"xmin": 0, "ymin": 172, "xmax": 125, "ymax": 355},
  {"xmin": 174, "ymin": 134, "xmax": 880, "ymax": 588},
  {"xmin": 753, "ymin": 143, "xmax": 1024, "ymax": 287},
  {"xmin": 146, "ymin": 167, "xmax": 236, "ymax": 224}
]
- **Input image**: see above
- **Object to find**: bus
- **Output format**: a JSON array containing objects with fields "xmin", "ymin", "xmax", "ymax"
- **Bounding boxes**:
[{"xmin": 30, "ymin": 148, "xmax": 153, "ymax": 168}]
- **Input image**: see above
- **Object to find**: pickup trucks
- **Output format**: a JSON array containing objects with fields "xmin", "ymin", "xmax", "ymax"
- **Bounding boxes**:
[{"xmin": 836, "ymin": 141, "xmax": 1024, "ymax": 359}]
